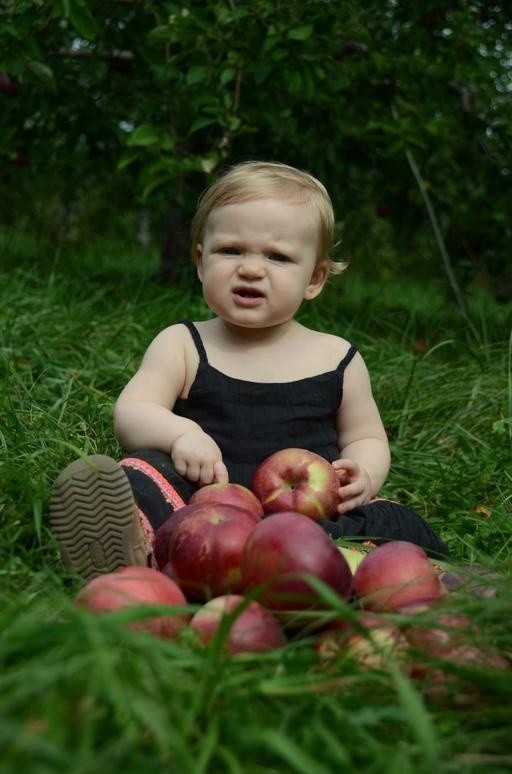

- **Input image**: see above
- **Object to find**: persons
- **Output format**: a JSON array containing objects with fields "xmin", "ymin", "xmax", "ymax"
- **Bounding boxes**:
[{"xmin": 48, "ymin": 157, "xmax": 449, "ymax": 580}]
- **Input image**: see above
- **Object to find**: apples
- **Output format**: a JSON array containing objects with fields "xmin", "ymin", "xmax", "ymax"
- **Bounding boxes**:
[{"xmin": 75, "ymin": 448, "xmax": 507, "ymax": 714}]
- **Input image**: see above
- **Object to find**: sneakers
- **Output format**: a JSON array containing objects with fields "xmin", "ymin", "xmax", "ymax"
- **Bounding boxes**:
[{"xmin": 49, "ymin": 455, "xmax": 152, "ymax": 589}]
